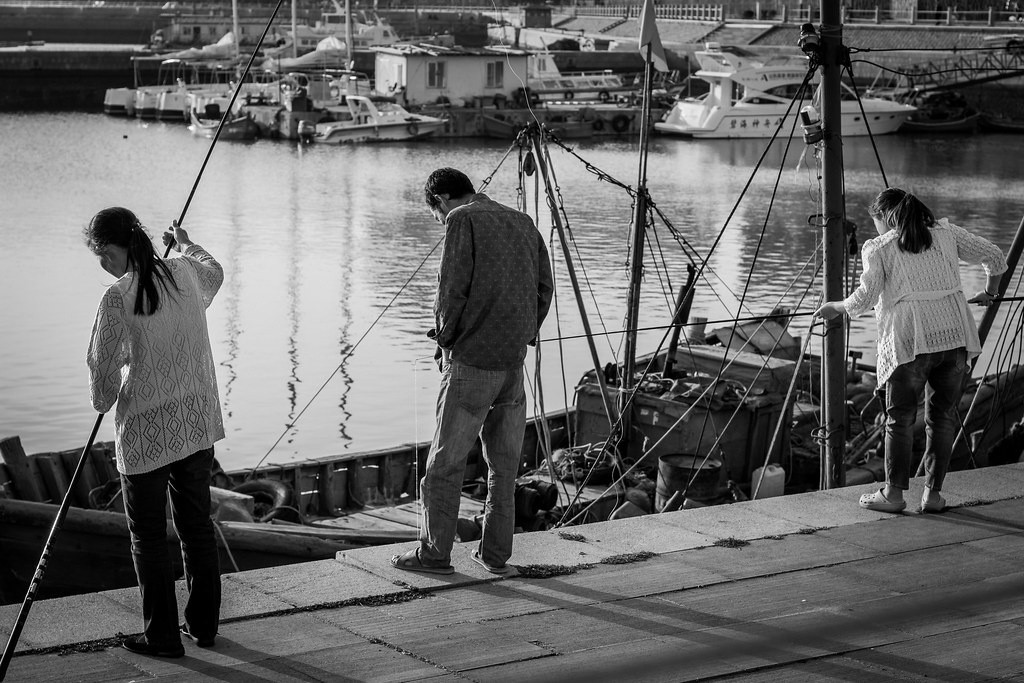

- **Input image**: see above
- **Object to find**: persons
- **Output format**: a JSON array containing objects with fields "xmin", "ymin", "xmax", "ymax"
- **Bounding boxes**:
[
  {"xmin": 84, "ymin": 207, "xmax": 224, "ymax": 659},
  {"xmin": 813, "ymin": 188, "xmax": 1009, "ymax": 512},
  {"xmin": 391, "ymin": 168, "xmax": 553, "ymax": 574}
]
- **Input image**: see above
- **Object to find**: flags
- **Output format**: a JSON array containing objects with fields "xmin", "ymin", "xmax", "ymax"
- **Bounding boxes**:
[{"xmin": 638, "ymin": 0, "xmax": 669, "ymax": 73}]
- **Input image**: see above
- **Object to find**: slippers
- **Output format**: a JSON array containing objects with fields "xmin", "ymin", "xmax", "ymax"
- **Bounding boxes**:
[
  {"xmin": 922, "ymin": 494, "xmax": 947, "ymax": 511},
  {"xmin": 390, "ymin": 547, "xmax": 456, "ymax": 575},
  {"xmin": 471, "ymin": 547, "xmax": 509, "ymax": 574},
  {"xmin": 859, "ymin": 488, "xmax": 907, "ymax": 512}
]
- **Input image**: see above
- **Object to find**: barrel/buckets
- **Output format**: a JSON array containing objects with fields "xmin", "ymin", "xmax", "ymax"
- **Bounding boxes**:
[
  {"xmin": 654, "ymin": 453, "xmax": 723, "ymax": 515},
  {"xmin": 751, "ymin": 463, "xmax": 785, "ymax": 500}
]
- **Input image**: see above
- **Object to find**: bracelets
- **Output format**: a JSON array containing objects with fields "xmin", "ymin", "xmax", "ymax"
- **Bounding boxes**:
[{"xmin": 985, "ymin": 290, "xmax": 999, "ymax": 297}]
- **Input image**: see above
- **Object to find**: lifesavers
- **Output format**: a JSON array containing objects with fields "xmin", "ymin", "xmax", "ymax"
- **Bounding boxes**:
[
  {"xmin": 598, "ymin": 91, "xmax": 609, "ymax": 100},
  {"xmin": 407, "ymin": 124, "xmax": 418, "ymax": 135},
  {"xmin": 594, "ymin": 119, "xmax": 603, "ymax": 131},
  {"xmin": 530, "ymin": 93, "xmax": 539, "ymax": 99},
  {"xmin": 565, "ymin": 91, "xmax": 573, "ymax": 98},
  {"xmin": 230, "ymin": 478, "xmax": 299, "ymax": 523},
  {"xmin": 612, "ymin": 115, "xmax": 630, "ymax": 133}
]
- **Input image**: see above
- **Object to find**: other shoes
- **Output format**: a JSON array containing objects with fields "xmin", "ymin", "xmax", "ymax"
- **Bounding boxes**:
[
  {"xmin": 123, "ymin": 636, "xmax": 185, "ymax": 658},
  {"xmin": 182, "ymin": 623, "xmax": 216, "ymax": 647}
]
